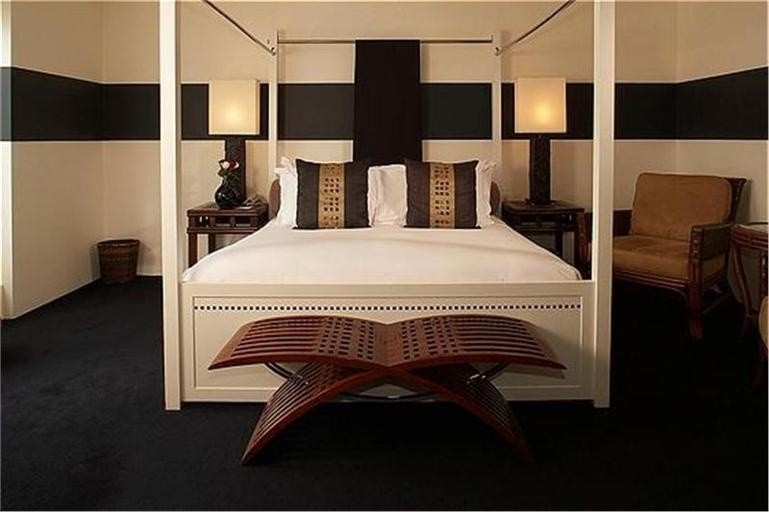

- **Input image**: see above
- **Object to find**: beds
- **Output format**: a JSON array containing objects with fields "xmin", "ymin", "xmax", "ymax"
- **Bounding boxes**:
[{"xmin": 158, "ymin": 1, "xmax": 617, "ymax": 413}]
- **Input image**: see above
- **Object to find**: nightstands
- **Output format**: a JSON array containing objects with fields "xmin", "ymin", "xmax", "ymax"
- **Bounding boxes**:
[
  {"xmin": 500, "ymin": 199, "xmax": 588, "ymax": 280},
  {"xmin": 186, "ymin": 201, "xmax": 269, "ymax": 269}
]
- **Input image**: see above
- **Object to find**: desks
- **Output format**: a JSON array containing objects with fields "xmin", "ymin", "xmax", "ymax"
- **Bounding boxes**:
[{"xmin": 729, "ymin": 222, "xmax": 769, "ymax": 358}]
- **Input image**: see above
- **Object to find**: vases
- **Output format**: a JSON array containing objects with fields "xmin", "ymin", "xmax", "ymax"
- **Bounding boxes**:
[{"xmin": 215, "ymin": 178, "xmax": 238, "ymax": 210}]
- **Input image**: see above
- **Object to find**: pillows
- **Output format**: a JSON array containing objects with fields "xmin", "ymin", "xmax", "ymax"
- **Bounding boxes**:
[
  {"xmin": 376, "ymin": 159, "xmax": 495, "ymax": 231},
  {"xmin": 272, "ymin": 154, "xmax": 376, "ymax": 231}
]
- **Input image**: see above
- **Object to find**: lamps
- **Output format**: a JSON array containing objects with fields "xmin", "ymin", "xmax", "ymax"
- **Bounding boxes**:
[
  {"xmin": 512, "ymin": 76, "xmax": 567, "ymax": 208},
  {"xmin": 207, "ymin": 77, "xmax": 260, "ymax": 206}
]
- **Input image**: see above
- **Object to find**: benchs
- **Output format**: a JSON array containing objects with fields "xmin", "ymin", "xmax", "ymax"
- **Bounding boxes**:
[{"xmin": 206, "ymin": 311, "xmax": 568, "ymax": 469}]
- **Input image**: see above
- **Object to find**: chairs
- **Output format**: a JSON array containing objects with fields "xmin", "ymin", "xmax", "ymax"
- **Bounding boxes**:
[{"xmin": 587, "ymin": 171, "xmax": 746, "ymax": 348}]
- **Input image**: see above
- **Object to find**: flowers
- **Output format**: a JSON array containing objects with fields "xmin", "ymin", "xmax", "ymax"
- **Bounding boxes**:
[{"xmin": 217, "ymin": 157, "xmax": 240, "ymax": 187}]
